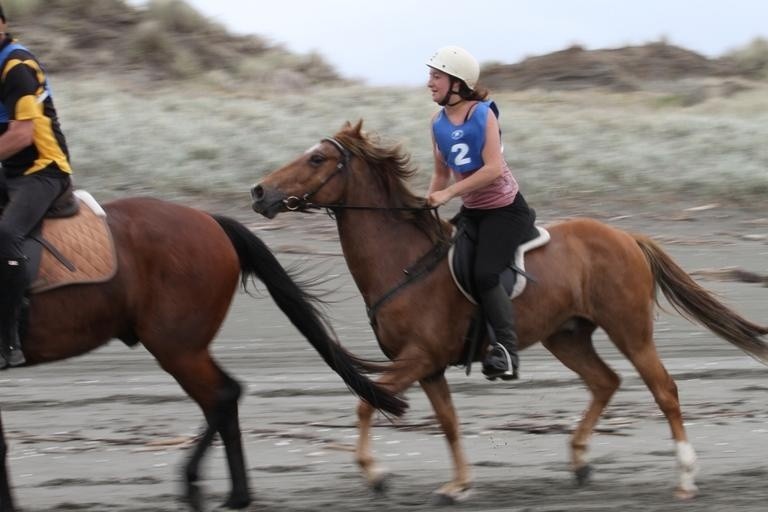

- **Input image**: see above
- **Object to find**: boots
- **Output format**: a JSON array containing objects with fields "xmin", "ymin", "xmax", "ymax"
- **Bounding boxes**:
[
  {"xmin": 475, "ymin": 280, "xmax": 522, "ymax": 381},
  {"xmin": 0, "ymin": 250, "xmax": 30, "ymax": 369}
]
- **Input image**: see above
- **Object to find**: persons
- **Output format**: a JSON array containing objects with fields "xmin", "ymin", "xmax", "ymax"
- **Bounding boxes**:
[
  {"xmin": 425, "ymin": 45, "xmax": 531, "ymax": 383},
  {"xmin": 0, "ymin": 4, "xmax": 75, "ymax": 374}
]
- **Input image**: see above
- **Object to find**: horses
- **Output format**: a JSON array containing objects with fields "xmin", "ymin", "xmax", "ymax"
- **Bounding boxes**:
[
  {"xmin": 0, "ymin": 196, "xmax": 415, "ymax": 512},
  {"xmin": 250, "ymin": 117, "xmax": 768, "ymax": 501}
]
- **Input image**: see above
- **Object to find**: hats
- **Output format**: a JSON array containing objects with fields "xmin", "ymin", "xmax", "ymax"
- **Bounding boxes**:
[{"xmin": 424, "ymin": 47, "xmax": 482, "ymax": 90}]
